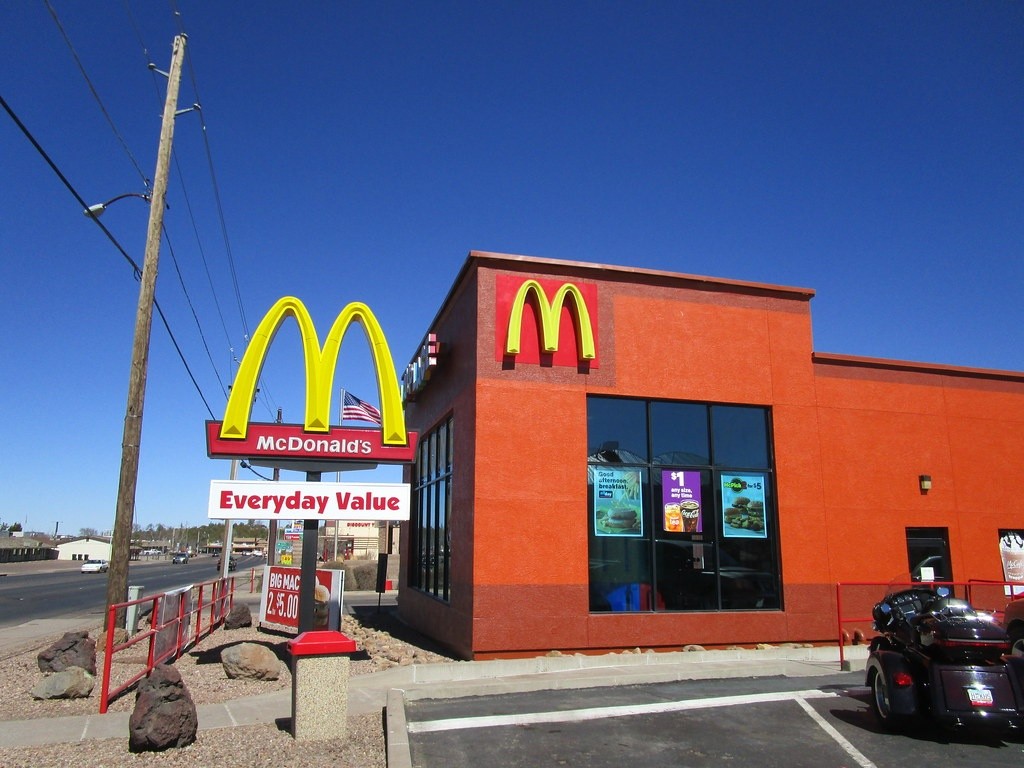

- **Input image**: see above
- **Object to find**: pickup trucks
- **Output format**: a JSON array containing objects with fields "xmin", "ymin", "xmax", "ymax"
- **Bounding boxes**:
[{"xmin": 139, "ymin": 549, "xmax": 162, "ymax": 555}]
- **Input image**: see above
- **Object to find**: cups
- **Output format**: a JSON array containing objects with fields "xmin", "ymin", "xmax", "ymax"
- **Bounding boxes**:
[
  {"xmin": 680, "ymin": 499, "xmax": 700, "ymax": 533},
  {"xmin": 999, "ymin": 542, "xmax": 1024, "ymax": 583},
  {"xmin": 664, "ymin": 502, "xmax": 684, "ymax": 533}
]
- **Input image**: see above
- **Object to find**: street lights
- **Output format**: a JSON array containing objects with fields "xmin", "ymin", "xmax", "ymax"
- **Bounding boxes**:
[{"xmin": 83, "ymin": 192, "xmax": 165, "ymax": 630}]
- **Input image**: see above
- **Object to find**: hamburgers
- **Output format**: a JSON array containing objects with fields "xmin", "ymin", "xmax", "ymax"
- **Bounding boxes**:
[
  {"xmin": 608, "ymin": 507, "xmax": 637, "ymax": 527},
  {"xmin": 723, "ymin": 497, "xmax": 763, "ymax": 529},
  {"xmin": 315, "ymin": 585, "xmax": 330, "ymax": 626}
]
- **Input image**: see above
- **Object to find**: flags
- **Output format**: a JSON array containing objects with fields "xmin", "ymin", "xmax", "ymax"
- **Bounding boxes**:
[{"xmin": 343, "ymin": 392, "xmax": 381, "ymax": 424}]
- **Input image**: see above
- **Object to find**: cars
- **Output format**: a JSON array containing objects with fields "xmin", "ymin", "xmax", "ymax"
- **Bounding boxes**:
[
  {"xmin": 1004, "ymin": 597, "xmax": 1023, "ymax": 658},
  {"xmin": 242, "ymin": 551, "xmax": 251, "ymax": 556},
  {"xmin": 212, "ymin": 553, "xmax": 219, "ymax": 557},
  {"xmin": 719, "ymin": 569, "xmax": 780, "ymax": 609},
  {"xmin": 81, "ymin": 560, "xmax": 108, "ymax": 574}
]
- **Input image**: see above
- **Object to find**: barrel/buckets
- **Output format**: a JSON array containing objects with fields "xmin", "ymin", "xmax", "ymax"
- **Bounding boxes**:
[{"xmin": 385, "ymin": 579, "xmax": 392, "ymax": 590}]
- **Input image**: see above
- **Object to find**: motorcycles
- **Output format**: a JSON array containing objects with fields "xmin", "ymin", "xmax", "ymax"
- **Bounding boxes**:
[{"xmin": 865, "ymin": 583, "xmax": 1023, "ymax": 736}]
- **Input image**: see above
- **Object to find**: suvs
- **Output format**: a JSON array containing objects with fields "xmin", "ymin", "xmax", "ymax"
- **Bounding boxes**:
[
  {"xmin": 217, "ymin": 555, "xmax": 237, "ymax": 571},
  {"xmin": 256, "ymin": 552, "xmax": 262, "ymax": 557},
  {"xmin": 173, "ymin": 553, "xmax": 188, "ymax": 564}
]
